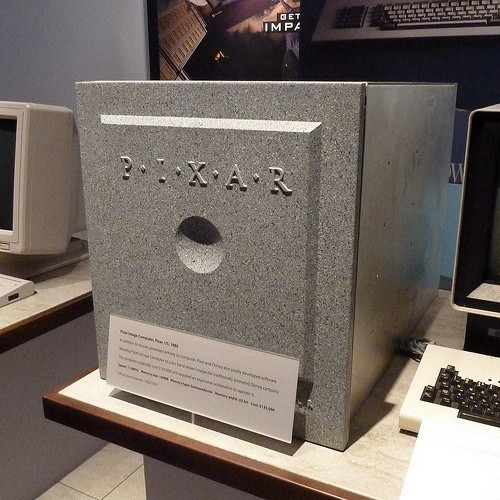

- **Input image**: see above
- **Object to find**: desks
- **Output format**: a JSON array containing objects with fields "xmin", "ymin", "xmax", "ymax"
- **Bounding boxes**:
[
  {"xmin": 0, "ymin": 236, "xmax": 109, "ymax": 499},
  {"xmin": 42, "ymin": 288, "xmax": 499, "ymax": 500}
]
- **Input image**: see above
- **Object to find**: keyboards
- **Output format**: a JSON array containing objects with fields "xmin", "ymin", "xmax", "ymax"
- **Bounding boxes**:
[
  {"xmin": 370, "ymin": 1, "xmax": 500, "ymax": 29},
  {"xmin": 0, "ymin": 273, "xmax": 35, "ymax": 308},
  {"xmin": 399, "ymin": 345, "xmax": 500, "ymax": 436}
]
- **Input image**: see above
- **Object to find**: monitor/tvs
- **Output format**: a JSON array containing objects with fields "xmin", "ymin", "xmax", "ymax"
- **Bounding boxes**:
[
  {"xmin": 452, "ymin": 106, "xmax": 500, "ymax": 320},
  {"xmin": 0, "ymin": 102, "xmax": 83, "ymax": 256}
]
